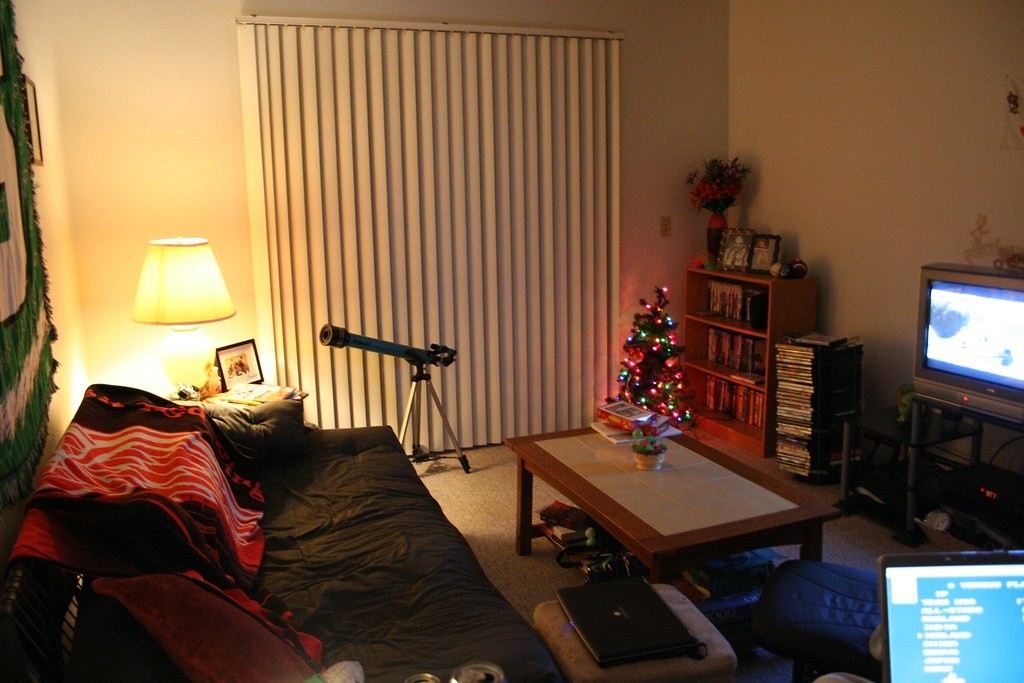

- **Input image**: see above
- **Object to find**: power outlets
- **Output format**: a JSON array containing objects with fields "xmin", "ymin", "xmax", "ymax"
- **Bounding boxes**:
[{"xmin": 660, "ymin": 215, "xmax": 673, "ymax": 237}]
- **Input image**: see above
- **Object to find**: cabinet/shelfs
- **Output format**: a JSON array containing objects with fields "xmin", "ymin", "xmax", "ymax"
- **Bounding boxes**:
[
  {"xmin": 683, "ymin": 267, "xmax": 821, "ymax": 456},
  {"xmin": 833, "ymin": 391, "xmax": 1023, "ymax": 547}
]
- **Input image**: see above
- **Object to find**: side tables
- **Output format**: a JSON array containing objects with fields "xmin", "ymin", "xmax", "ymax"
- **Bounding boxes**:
[{"xmin": 165, "ymin": 385, "xmax": 309, "ymax": 412}]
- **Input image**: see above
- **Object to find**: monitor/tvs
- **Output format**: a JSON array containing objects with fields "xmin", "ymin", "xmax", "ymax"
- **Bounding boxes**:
[{"xmin": 913, "ymin": 261, "xmax": 1024, "ymax": 421}]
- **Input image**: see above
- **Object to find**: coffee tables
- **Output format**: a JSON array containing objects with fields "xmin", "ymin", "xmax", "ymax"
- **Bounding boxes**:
[{"xmin": 501, "ymin": 419, "xmax": 844, "ymax": 587}]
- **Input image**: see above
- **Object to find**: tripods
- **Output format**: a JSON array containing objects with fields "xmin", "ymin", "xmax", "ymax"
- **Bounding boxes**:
[{"xmin": 399, "ymin": 362, "xmax": 472, "ymax": 474}]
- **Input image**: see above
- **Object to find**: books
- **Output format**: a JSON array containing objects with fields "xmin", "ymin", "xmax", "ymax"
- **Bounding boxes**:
[
  {"xmin": 540, "ymin": 499, "xmax": 592, "ymax": 543},
  {"xmin": 774, "ymin": 331, "xmax": 865, "ymax": 478},
  {"xmin": 706, "ymin": 327, "xmax": 766, "ymax": 376},
  {"xmin": 705, "ymin": 374, "xmax": 766, "ymax": 430},
  {"xmin": 706, "ymin": 276, "xmax": 763, "ymax": 323}
]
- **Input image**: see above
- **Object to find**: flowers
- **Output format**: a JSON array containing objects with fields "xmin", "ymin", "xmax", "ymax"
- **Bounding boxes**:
[{"xmin": 685, "ymin": 154, "xmax": 749, "ymax": 213}]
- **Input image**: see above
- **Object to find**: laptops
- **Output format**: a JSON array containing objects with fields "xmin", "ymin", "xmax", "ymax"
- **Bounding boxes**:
[
  {"xmin": 555, "ymin": 576, "xmax": 700, "ymax": 668},
  {"xmin": 878, "ymin": 548, "xmax": 1024, "ymax": 683}
]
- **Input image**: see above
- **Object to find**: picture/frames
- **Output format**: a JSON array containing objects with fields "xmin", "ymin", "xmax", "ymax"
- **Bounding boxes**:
[
  {"xmin": 217, "ymin": 339, "xmax": 263, "ymax": 389},
  {"xmin": 20, "ymin": 74, "xmax": 46, "ymax": 164},
  {"xmin": 751, "ymin": 234, "xmax": 783, "ymax": 273},
  {"xmin": 718, "ymin": 229, "xmax": 755, "ymax": 271}
]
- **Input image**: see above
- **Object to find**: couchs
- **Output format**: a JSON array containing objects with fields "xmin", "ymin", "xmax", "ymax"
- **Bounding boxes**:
[{"xmin": 1, "ymin": 384, "xmax": 565, "ymax": 682}]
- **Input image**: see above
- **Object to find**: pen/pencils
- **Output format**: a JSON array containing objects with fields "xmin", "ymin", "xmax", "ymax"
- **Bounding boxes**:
[{"xmin": 226, "ymin": 398, "xmax": 263, "ymax": 408}]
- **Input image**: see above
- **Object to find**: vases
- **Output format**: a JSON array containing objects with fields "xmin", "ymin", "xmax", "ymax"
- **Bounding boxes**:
[{"xmin": 705, "ymin": 205, "xmax": 726, "ymax": 263}]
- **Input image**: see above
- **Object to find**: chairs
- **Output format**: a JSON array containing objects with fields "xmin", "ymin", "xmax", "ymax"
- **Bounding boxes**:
[{"xmin": 753, "ymin": 558, "xmax": 881, "ymax": 683}]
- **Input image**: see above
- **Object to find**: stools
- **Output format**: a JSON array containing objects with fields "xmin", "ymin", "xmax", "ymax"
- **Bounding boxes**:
[{"xmin": 535, "ymin": 584, "xmax": 740, "ymax": 683}]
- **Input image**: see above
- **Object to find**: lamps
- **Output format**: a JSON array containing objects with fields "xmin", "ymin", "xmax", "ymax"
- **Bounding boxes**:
[{"xmin": 133, "ymin": 238, "xmax": 233, "ymax": 391}]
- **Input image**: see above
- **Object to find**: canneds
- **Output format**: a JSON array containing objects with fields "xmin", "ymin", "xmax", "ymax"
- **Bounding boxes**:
[{"xmin": 403, "ymin": 659, "xmax": 508, "ymax": 683}]
